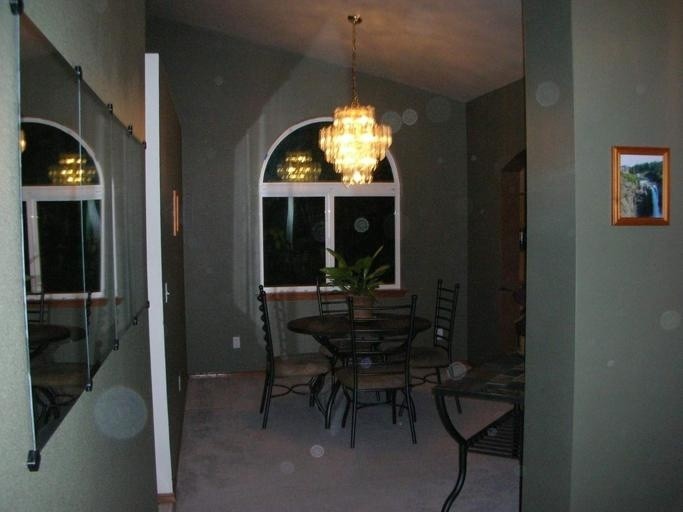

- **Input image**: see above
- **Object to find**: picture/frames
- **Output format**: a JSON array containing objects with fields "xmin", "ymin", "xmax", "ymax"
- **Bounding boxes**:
[{"xmin": 609, "ymin": 144, "xmax": 671, "ymax": 227}]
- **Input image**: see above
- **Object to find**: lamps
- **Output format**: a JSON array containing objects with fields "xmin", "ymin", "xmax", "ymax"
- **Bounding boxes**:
[
  {"xmin": 273, "ymin": 150, "xmax": 321, "ymax": 183},
  {"xmin": 19, "ymin": 120, "xmax": 32, "ymax": 155},
  {"xmin": 317, "ymin": 15, "xmax": 396, "ymax": 187},
  {"xmin": 48, "ymin": 145, "xmax": 96, "ymax": 185}
]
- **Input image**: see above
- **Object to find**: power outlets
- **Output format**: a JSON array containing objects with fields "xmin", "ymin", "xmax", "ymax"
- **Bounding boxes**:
[{"xmin": 232, "ymin": 337, "xmax": 242, "ymax": 350}]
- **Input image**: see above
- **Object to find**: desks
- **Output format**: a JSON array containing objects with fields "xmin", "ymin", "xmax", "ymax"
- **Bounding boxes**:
[
  {"xmin": 429, "ymin": 350, "xmax": 523, "ymax": 511},
  {"xmin": 291, "ymin": 311, "xmax": 427, "ymax": 427}
]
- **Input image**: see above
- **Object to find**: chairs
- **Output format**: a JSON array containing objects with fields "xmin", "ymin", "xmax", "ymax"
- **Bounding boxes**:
[
  {"xmin": 389, "ymin": 277, "xmax": 461, "ymax": 416},
  {"xmin": 252, "ymin": 284, "xmax": 335, "ymax": 430},
  {"xmin": 335, "ymin": 293, "xmax": 419, "ymax": 449},
  {"xmin": 311, "ymin": 279, "xmax": 372, "ymax": 419}
]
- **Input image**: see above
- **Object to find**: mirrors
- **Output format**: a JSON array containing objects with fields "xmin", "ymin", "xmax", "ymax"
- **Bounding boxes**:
[{"xmin": 12, "ymin": 5, "xmax": 147, "ymax": 467}]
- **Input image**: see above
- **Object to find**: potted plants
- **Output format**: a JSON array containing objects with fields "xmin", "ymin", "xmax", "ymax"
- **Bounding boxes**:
[{"xmin": 317, "ymin": 244, "xmax": 394, "ymax": 320}]
